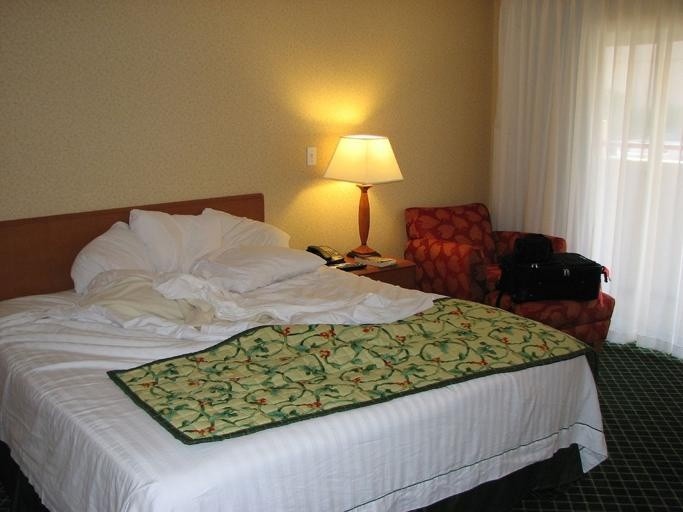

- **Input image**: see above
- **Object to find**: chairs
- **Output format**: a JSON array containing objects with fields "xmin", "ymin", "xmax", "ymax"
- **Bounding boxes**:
[{"xmin": 404, "ymin": 202, "xmax": 615, "ymax": 354}]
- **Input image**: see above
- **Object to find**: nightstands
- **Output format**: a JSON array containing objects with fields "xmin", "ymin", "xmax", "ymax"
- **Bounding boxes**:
[{"xmin": 341, "ymin": 254, "xmax": 417, "ymax": 290}]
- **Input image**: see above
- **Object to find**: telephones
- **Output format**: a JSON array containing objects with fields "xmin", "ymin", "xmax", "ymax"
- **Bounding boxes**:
[{"xmin": 306, "ymin": 245, "xmax": 344, "ymax": 265}]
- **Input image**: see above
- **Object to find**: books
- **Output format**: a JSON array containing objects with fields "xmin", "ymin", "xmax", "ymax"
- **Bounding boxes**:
[{"xmin": 359, "ymin": 256, "xmax": 396, "ymax": 268}]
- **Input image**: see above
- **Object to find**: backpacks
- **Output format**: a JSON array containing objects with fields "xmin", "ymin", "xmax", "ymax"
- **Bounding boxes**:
[{"xmin": 496, "ymin": 233, "xmax": 605, "ymax": 304}]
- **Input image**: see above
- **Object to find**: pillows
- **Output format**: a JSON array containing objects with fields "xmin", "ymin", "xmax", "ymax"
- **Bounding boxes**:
[{"xmin": 71, "ymin": 208, "xmax": 327, "ymax": 293}]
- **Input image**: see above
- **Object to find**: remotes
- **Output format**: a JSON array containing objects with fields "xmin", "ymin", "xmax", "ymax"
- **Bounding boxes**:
[{"xmin": 336, "ymin": 263, "xmax": 367, "ymax": 271}]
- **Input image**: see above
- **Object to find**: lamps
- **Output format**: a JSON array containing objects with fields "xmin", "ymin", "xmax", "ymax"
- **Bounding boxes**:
[{"xmin": 321, "ymin": 134, "xmax": 404, "ymax": 258}]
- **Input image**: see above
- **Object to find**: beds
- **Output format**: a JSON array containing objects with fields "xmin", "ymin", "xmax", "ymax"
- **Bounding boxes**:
[{"xmin": 0, "ymin": 192, "xmax": 609, "ymax": 511}]
